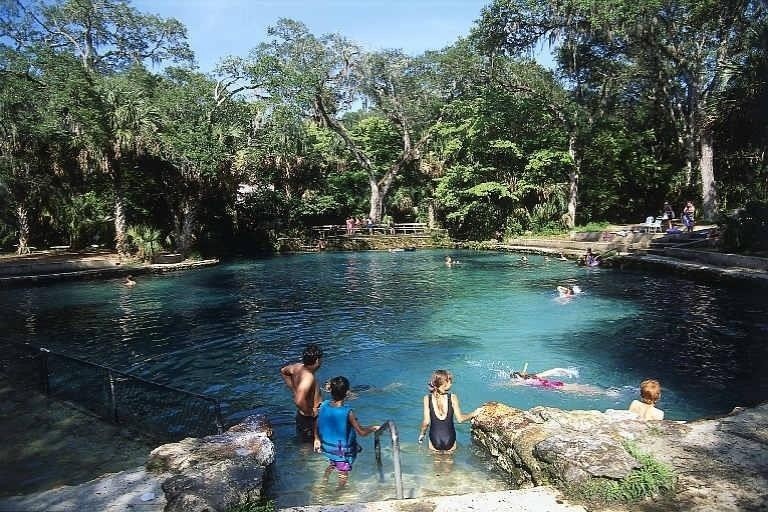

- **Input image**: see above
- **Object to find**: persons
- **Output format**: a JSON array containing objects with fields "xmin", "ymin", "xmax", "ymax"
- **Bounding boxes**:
[
  {"xmin": 680, "ymin": 201, "xmax": 696, "ymax": 233},
  {"xmin": 502, "ymin": 360, "xmax": 617, "ymax": 396},
  {"xmin": 662, "ymin": 201, "xmax": 674, "ymax": 229},
  {"xmin": 312, "ymin": 375, "xmax": 382, "ymax": 493},
  {"xmin": 630, "ymin": 376, "xmax": 664, "ymax": 420},
  {"xmin": 345, "ymin": 212, "xmax": 373, "ymax": 236},
  {"xmin": 556, "ymin": 284, "xmax": 575, "ymax": 303},
  {"xmin": 582, "ymin": 247, "xmax": 599, "ymax": 267},
  {"xmin": 415, "ymin": 367, "xmax": 482, "ymax": 455},
  {"xmin": 280, "ymin": 342, "xmax": 324, "ymax": 443},
  {"xmin": 445, "ymin": 255, "xmax": 452, "ymax": 267}
]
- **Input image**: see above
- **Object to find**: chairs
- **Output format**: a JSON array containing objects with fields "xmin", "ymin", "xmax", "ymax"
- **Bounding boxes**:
[{"xmin": 639, "ymin": 216, "xmax": 664, "ymax": 234}]
